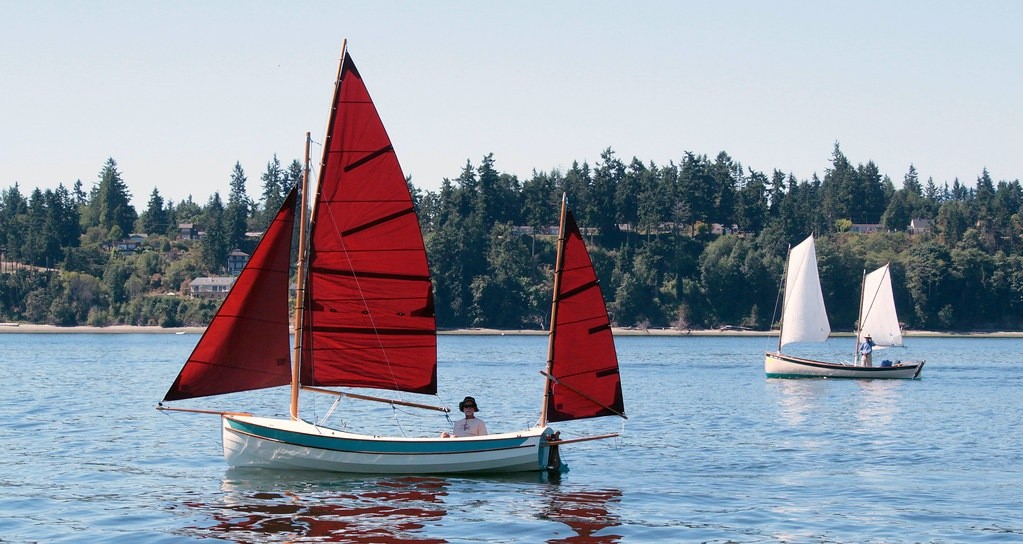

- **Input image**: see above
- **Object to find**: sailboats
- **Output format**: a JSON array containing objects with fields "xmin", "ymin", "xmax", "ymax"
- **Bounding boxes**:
[
  {"xmin": 761, "ymin": 235, "xmax": 926, "ymax": 380},
  {"xmin": 152, "ymin": 34, "xmax": 629, "ymax": 478}
]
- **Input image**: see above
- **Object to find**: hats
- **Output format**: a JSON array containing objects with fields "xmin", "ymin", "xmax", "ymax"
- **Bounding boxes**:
[
  {"xmin": 864, "ymin": 334, "xmax": 872, "ymax": 338},
  {"xmin": 459, "ymin": 396, "xmax": 479, "ymax": 413}
]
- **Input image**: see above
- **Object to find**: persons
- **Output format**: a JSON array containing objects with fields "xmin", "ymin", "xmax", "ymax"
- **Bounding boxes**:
[
  {"xmin": 440, "ymin": 396, "xmax": 488, "ymax": 438},
  {"xmin": 859, "ymin": 334, "xmax": 876, "ymax": 367}
]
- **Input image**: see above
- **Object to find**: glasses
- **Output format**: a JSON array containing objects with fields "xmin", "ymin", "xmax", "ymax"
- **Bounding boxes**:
[{"xmin": 463, "ymin": 405, "xmax": 475, "ymax": 408}]
